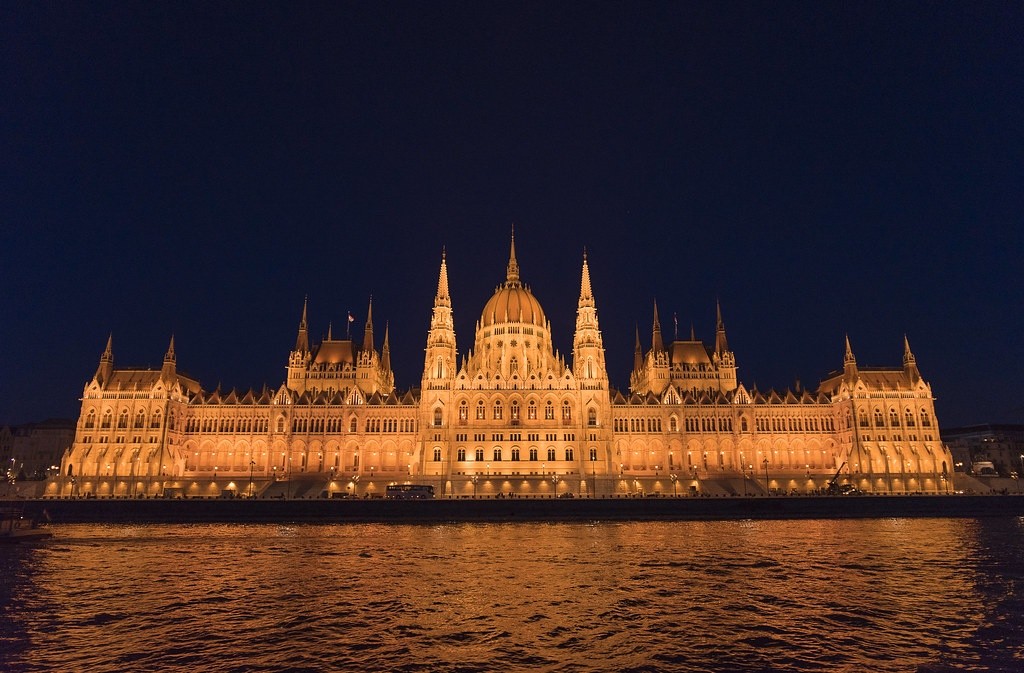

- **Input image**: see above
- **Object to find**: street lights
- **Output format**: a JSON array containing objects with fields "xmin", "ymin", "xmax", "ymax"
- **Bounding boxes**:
[
  {"xmin": 591, "ymin": 456, "xmax": 597, "ymax": 498},
  {"xmin": 133, "ymin": 457, "xmax": 141, "ymax": 499},
  {"xmin": 669, "ymin": 472, "xmax": 679, "ymax": 498},
  {"xmin": 470, "ymin": 474, "xmax": 479, "ymax": 499},
  {"xmin": 741, "ymin": 456, "xmax": 748, "ymax": 497},
  {"xmin": 550, "ymin": 473, "xmax": 560, "ymax": 499},
  {"xmin": 440, "ymin": 457, "xmax": 444, "ymax": 497},
  {"xmin": 10, "ymin": 458, "xmax": 15, "ymax": 475},
  {"xmin": 349, "ymin": 473, "xmax": 360, "ymax": 501},
  {"xmin": 940, "ymin": 472, "xmax": 950, "ymax": 495},
  {"xmin": 760, "ymin": 458, "xmax": 772, "ymax": 498},
  {"xmin": 249, "ymin": 461, "xmax": 257, "ymax": 498},
  {"xmin": 69, "ymin": 476, "xmax": 76, "ymax": 499},
  {"xmin": 287, "ymin": 457, "xmax": 292, "ymax": 500},
  {"xmin": 1009, "ymin": 471, "xmax": 1022, "ymax": 494}
]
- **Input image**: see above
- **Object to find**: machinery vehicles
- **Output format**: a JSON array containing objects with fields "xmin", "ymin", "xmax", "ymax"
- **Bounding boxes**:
[{"xmin": 827, "ymin": 460, "xmax": 858, "ymax": 496}]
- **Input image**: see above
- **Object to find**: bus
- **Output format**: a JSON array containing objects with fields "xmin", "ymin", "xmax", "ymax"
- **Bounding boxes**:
[{"xmin": 384, "ymin": 484, "xmax": 436, "ymax": 500}]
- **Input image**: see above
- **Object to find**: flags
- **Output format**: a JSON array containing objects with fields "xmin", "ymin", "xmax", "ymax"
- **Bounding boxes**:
[{"xmin": 349, "ymin": 313, "xmax": 354, "ymax": 322}]
